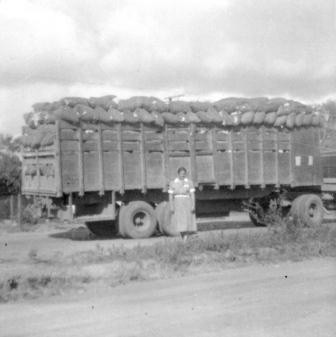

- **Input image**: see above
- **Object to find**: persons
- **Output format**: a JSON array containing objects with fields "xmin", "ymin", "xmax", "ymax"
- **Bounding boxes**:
[{"xmin": 168, "ymin": 167, "xmax": 196, "ymax": 242}]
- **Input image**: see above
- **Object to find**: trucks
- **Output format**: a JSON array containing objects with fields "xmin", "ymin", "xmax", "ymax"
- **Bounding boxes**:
[{"xmin": 21, "ymin": 120, "xmax": 336, "ymax": 239}]
